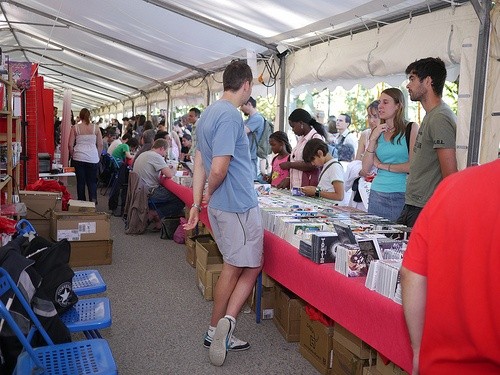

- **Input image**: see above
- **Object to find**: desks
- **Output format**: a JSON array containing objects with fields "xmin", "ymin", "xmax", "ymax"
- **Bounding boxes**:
[{"xmin": 128, "ymin": 153, "xmax": 413, "ymax": 375}]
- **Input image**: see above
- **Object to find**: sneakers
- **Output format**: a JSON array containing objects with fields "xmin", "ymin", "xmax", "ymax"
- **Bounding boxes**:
[{"xmin": 203, "ymin": 318, "xmax": 251, "ymax": 367}]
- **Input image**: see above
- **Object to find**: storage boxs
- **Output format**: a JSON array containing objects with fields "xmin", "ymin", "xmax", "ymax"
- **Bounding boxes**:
[
  {"xmin": 18, "ymin": 190, "xmax": 63, "ymax": 220},
  {"xmin": 50, "ymin": 210, "xmax": 111, "ymax": 243},
  {"xmin": 49, "ymin": 237, "xmax": 113, "ymax": 266}
]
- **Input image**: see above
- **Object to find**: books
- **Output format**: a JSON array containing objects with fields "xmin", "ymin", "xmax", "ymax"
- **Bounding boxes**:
[{"xmin": 171, "ymin": 157, "xmax": 414, "ymax": 312}]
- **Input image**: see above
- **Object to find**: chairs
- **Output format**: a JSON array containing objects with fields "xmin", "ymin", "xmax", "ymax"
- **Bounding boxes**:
[
  {"xmin": 59, "ymin": 297, "xmax": 111, "ymax": 341},
  {"xmin": 14, "ymin": 217, "xmax": 107, "ymax": 296},
  {"xmin": 0, "ymin": 267, "xmax": 119, "ymax": 374}
]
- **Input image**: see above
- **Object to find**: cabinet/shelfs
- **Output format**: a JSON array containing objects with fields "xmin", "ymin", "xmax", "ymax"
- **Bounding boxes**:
[{"xmin": 0, "ymin": 49, "xmax": 23, "ymax": 208}]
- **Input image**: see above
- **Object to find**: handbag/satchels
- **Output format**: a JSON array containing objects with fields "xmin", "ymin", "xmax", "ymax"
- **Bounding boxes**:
[
  {"xmin": 159, "ymin": 215, "xmax": 181, "ymax": 241},
  {"xmin": 301, "ymin": 166, "xmax": 325, "ymax": 190}
]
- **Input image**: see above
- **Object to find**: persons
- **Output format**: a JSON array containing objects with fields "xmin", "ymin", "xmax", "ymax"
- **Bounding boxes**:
[
  {"xmin": 401, "ymin": 149, "xmax": 500, "ymax": 375},
  {"xmin": 279, "ymin": 109, "xmax": 328, "ymax": 190},
  {"xmin": 362, "ymin": 88, "xmax": 420, "ymax": 226},
  {"xmin": 261, "ymin": 131, "xmax": 291, "ymax": 188},
  {"xmin": 349, "ymin": 99, "xmax": 386, "ymax": 213},
  {"xmin": 68, "ymin": 108, "xmax": 103, "ymax": 208},
  {"xmin": 131, "ymin": 140, "xmax": 183, "ymax": 222},
  {"xmin": 240, "ymin": 96, "xmax": 264, "ymax": 183},
  {"xmin": 331, "ymin": 113, "xmax": 358, "ymax": 159},
  {"xmin": 181, "ymin": 60, "xmax": 264, "ymax": 365},
  {"xmin": 51, "ymin": 103, "xmax": 201, "ymax": 167},
  {"xmin": 387, "ymin": 56, "xmax": 457, "ymax": 242},
  {"xmin": 300, "ymin": 138, "xmax": 344, "ymax": 204}
]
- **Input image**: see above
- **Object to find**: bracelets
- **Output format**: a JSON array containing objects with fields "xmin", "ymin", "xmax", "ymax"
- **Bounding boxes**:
[{"xmin": 365, "ymin": 148, "xmax": 376, "ymax": 154}]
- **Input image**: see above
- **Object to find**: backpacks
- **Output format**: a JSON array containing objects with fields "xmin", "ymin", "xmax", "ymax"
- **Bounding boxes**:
[
  {"xmin": 23, "ymin": 234, "xmax": 78, "ymax": 316},
  {"xmin": 250, "ymin": 113, "xmax": 274, "ymax": 159}
]
